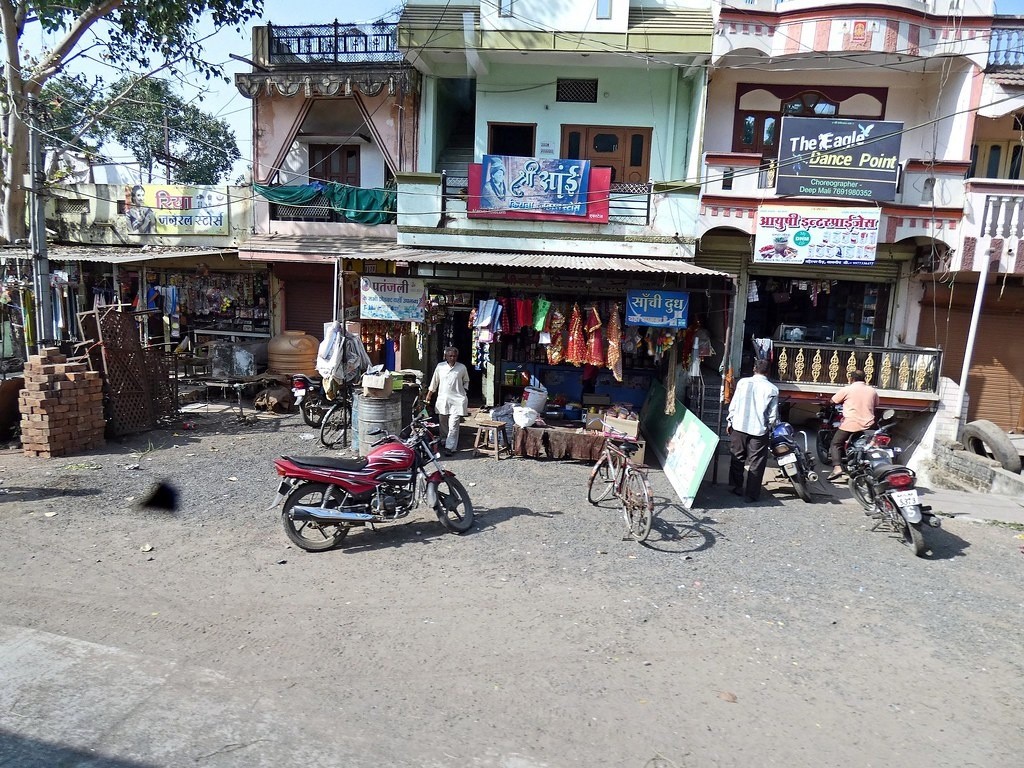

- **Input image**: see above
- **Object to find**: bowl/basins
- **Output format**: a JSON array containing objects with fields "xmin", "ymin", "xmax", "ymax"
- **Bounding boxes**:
[
  {"xmin": 545, "ymin": 412, "xmax": 564, "ymax": 419},
  {"xmin": 558, "ymin": 406, "xmax": 581, "ymax": 419}
]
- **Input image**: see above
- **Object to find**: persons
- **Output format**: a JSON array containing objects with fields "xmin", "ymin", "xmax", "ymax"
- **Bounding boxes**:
[
  {"xmin": 481, "ymin": 169, "xmax": 508, "ymax": 209},
  {"xmin": 826, "ymin": 370, "xmax": 880, "ymax": 480},
  {"xmin": 126, "ymin": 185, "xmax": 156, "ymax": 234},
  {"xmin": 426, "ymin": 347, "xmax": 470, "ymax": 457},
  {"xmin": 726, "ymin": 359, "xmax": 780, "ymax": 503}
]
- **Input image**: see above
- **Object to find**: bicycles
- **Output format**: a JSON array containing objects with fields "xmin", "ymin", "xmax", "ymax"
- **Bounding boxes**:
[
  {"xmin": 319, "ymin": 384, "xmax": 353, "ymax": 446},
  {"xmin": 585, "ymin": 416, "xmax": 654, "ymax": 544}
]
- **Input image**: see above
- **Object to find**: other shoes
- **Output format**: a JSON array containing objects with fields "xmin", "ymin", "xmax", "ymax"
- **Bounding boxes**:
[
  {"xmin": 729, "ymin": 485, "xmax": 743, "ymax": 496},
  {"xmin": 442, "ymin": 443, "xmax": 447, "ymax": 453},
  {"xmin": 746, "ymin": 494, "xmax": 755, "ymax": 502},
  {"xmin": 446, "ymin": 449, "xmax": 454, "ymax": 457}
]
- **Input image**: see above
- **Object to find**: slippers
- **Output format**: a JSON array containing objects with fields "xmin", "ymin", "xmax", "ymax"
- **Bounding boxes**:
[{"xmin": 826, "ymin": 470, "xmax": 846, "ymax": 480}]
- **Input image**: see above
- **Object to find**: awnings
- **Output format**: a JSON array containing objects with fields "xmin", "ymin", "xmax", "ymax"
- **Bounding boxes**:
[{"xmin": 325, "ymin": 247, "xmax": 730, "ymax": 278}]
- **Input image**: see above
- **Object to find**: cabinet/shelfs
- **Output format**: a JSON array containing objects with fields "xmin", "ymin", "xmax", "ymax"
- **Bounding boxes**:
[{"xmin": 841, "ymin": 281, "xmax": 888, "ymax": 346}]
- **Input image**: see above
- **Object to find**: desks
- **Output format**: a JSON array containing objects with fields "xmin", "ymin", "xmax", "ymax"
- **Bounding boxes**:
[
  {"xmin": 512, "ymin": 420, "xmax": 645, "ymax": 466},
  {"xmin": 498, "ymin": 383, "xmax": 526, "ymax": 405},
  {"xmin": 192, "ymin": 318, "xmax": 270, "ymax": 374}
]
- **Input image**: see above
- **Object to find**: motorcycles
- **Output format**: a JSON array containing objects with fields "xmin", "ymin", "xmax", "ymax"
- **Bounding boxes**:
[
  {"xmin": 264, "ymin": 388, "xmax": 476, "ymax": 554},
  {"xmin": 841, "ymin": 409, "xmax": 941, "ymax": 556},
  {"xmin": 292, "ymin": 373, "xmax": 353, "ymax": 429},
  {"xmin": 810, "ymin": 395, "xmax": 893, "ymax": 465},
  {"xmin": 767, "ymin": 395, "xmax": 819, "ymax": 503}
]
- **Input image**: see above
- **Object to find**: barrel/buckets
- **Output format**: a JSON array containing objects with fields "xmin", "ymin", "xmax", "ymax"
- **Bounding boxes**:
[{"xmin": 350, "ymin": 388, "xmax": 413, "ymax": 458}]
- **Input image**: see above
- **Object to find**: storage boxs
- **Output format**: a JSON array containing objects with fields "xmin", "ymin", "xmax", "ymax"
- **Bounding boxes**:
[
  {"xmin": 582, "ymin": 393, "xmax": 610, "ymax": 405},
  {"xmin": 603, "ymin": 412, "xmax": 640, "ymax": 441},
  {"xmin": 361, "ymin": 369, "xmax": 405, "ymax": 397},
  {"xmin": 585, "ymin": 409, "xmax": 604, "ymax": 431}
]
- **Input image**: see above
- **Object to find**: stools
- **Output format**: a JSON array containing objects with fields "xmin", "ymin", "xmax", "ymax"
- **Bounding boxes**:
[{"xmin": 471, "ymin": 419, "xmax": 512, "ymax": 461}]
